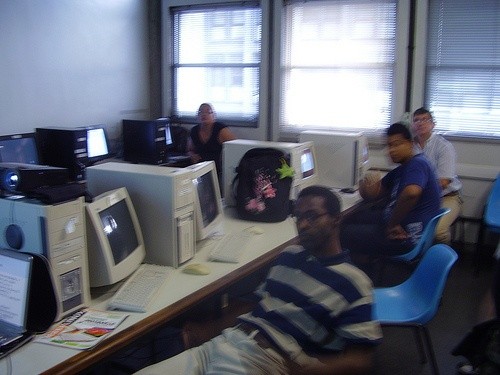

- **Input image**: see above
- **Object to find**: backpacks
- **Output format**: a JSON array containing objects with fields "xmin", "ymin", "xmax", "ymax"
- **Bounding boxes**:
[{"xmin": 230, "ymin": 147, "xmax": 293, "ymax": 224}]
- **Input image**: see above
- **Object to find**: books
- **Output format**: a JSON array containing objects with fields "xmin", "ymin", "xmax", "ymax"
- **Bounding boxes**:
[{"xmin": 33, "ymin": 307, "xmax": 130, "ymax": 350}]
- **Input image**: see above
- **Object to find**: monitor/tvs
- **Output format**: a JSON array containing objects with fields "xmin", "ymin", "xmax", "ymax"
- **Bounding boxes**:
[
  {"xmin": 0, "ymin": 133, "xmax": 42, "ymax": 166},
  {"xmin": 86, "ymin": 119, "xmax": 370, "ymax": 290}
]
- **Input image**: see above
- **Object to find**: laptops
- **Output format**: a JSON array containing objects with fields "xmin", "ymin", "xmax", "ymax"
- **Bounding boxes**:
[{"xmin": 0, "ymin": 249, "xmax": 33, "ymax": 353}]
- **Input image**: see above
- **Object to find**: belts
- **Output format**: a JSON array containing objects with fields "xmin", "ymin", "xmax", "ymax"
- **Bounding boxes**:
[{"xmin": 238, "ymin": 320, "xmax": 274, "ymax": 352}]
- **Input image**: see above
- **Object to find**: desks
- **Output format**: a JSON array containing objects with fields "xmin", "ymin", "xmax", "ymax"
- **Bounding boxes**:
[{"xmin": 0, "ymin": 171, "xmax": 387, "ymax": 375}]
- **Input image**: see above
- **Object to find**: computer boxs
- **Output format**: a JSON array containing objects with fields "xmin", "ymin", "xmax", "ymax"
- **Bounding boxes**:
[
  {"xmin": 298, "ymin": 129, "xmax": 367, "ymax": 190},
  {"xmin": 36, "ymin": 127, "xmax": 90, "ymax": 180},
  {"xmin": 222, "ymin": 139, "xmax": 301, "ymax": 208},
  {"xmin": 85, "ymin": 162, "xmax": 197, "ymax": 268},
  {"xmin": 122, "ymin": 119, "xmax": 167, "ymax": 164},
  {"xmin": 0, "ymin": 195, "xmax": 93, "ymax": 322}
]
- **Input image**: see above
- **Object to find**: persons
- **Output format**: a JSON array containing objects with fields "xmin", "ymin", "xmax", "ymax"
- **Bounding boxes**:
[
  {"xmin": 131, "ymin": 185, "xmax": 383, "ymax": 375},
  {"xmin": 397, "ymin": 109, "xmax": 464, "ymax": 249},
  {"xmin": 186, "ymin": 103, "xmax": 237, "ymax": 178},
  {"xmin": 340, "ymin": 122, "xmax": 441, "ymax": 256}
]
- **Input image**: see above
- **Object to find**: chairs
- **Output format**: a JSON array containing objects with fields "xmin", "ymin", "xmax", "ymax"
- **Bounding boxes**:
[{"xmin": 372, "ymin": 172, "xmax": 500, "ymax": 375}]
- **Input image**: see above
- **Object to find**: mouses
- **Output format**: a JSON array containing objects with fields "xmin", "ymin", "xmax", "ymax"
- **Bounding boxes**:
[
  {"xmin": 246, "ymin": 227, "xmax": 263, "ymax": 234},
  {"xmin": 182, "ymin": 264, "xmax": 210, "ymax": 275}
]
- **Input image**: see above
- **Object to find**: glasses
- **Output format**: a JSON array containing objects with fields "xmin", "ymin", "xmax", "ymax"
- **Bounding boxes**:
[
  {"xmin": 414, "ymin": 116, "xmax": 431, "ymax": 123},
  {"xmin": 291, "ymin": 209, "xmax": 334, "ymax": 224}
]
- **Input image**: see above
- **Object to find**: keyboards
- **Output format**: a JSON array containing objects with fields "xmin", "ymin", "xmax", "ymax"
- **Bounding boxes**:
[
  {"xmin": 105, "ymin": 263, "xmax": 173, "ymax": 313},
  {"xmin": 208, "ymin": 233, "xmax": 249, "ymax": 264}
]
